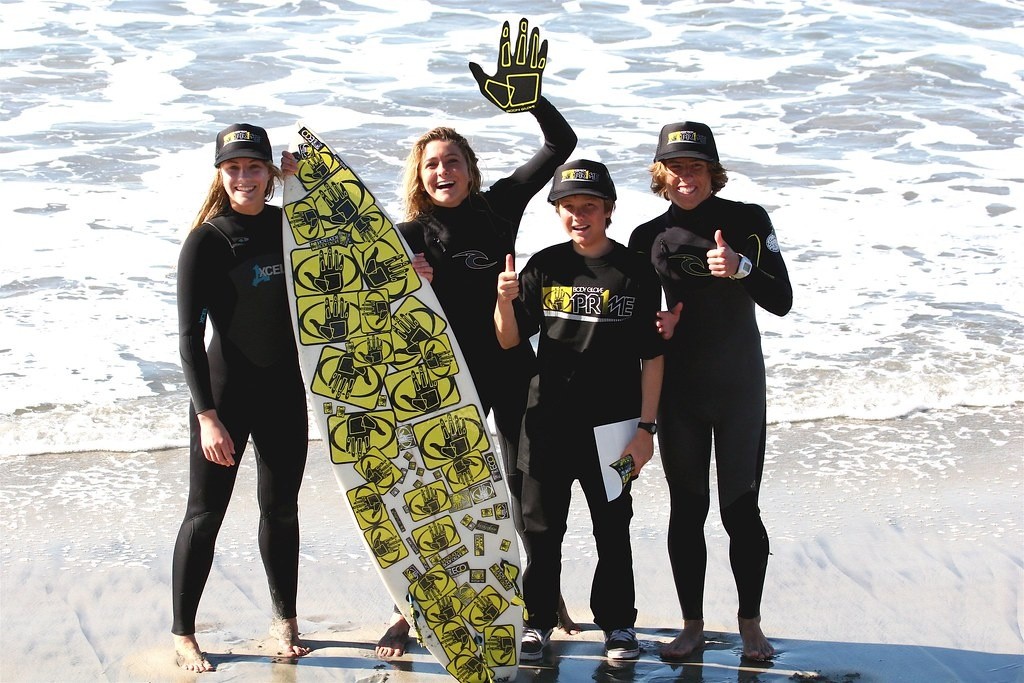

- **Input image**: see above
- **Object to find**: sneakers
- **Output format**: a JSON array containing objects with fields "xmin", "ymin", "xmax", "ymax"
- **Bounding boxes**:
[
  {"xmin": 520, "ymin": 626, "xmax": 554, "ymax": 660},
  {"xmin": 603, "ymin": 626, "xmax": 640, "ymax": 659}
]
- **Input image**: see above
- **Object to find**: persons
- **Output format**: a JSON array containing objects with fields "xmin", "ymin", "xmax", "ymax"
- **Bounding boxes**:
[
  {"xmin": 170, "ymin": 122, "xmax": 311, "ymax": 673},
  {"xmin": 629, "ymin": 121, "xmax": 792, "ymax": 660},
  {"xmin": 280, "ymin": 17, "xmax": 578, "ymax": 657},
  {"xmin": 496, "ymin": 159, "xmax": 665, "ymax": 658}
]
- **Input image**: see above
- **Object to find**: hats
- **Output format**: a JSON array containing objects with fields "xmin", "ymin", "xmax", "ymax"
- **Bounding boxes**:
[
  {"xmin": 546, "ymin": 159, "xmax": 617, "ymax": 202},
  {"xmin": 214, "ymin": 123, "xmax": 272, "ymax": 162},
  {"xmin": 654, "ymin": 121, "xmax": 720, "ymax": 165}
]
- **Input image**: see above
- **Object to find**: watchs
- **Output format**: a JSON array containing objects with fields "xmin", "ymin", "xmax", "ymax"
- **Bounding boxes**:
[
  {"xmin": 637, "ymin": 422, "xmax": 657, "ymax": 434},
  {"xmin": 729, "ymin": 252, "xmax": 751, "ymax": 281}
]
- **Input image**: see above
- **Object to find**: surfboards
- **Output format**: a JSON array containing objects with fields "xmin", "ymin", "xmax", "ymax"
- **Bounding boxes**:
[{"xmin": 282, "ymin": 120, "xmax": 524, "ymax": 683}]
